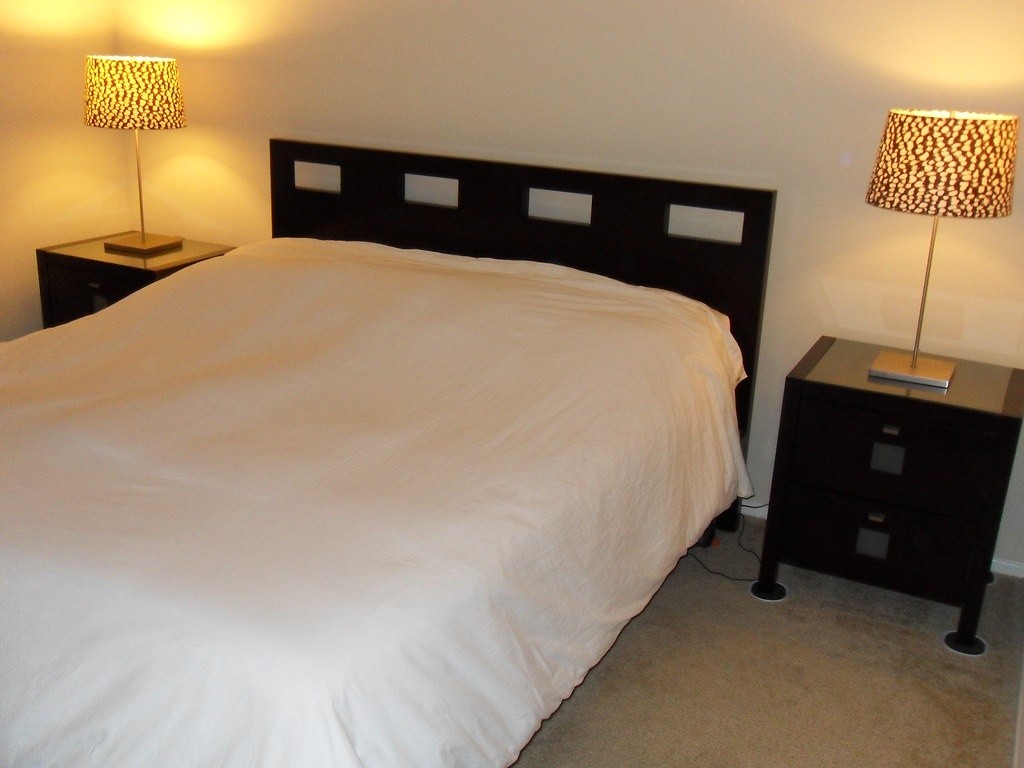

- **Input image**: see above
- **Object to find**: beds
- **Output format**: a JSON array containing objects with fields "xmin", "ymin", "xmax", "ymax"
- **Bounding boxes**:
[{"xmin": 0, "ymin": 142, "xmax": 775, "ymax": 768}]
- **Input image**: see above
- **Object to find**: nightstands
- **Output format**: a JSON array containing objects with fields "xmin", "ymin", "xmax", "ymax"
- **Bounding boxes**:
[
  {"xmin": 36, "ymin": 230, "xmax": 237, "ymax": 344},
  {"xmin": 752, "ymin": 337, "xmax": 1024, "ymax": 659}
]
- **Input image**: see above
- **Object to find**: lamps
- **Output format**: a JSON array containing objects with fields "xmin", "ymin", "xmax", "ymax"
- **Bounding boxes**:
[
  {"xmin": 82, "ymin": 54, "xmax": 186, "ymax": 257},
  {"xmin": 866, "ymin": 109, "xmax": 1018, "ymax": 387}
]
